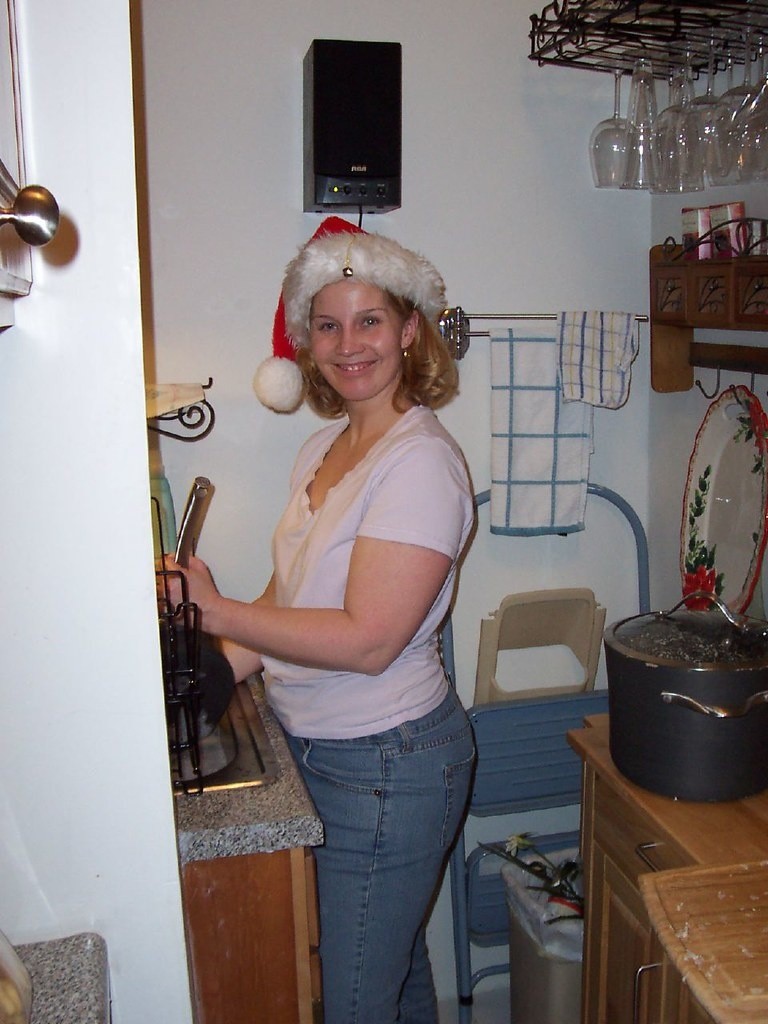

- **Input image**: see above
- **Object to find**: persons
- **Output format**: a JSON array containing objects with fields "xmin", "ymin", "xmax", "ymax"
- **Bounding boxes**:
[{"xmin": 155, "ymin": 216, "xmax": 478, "ymax": 1023}]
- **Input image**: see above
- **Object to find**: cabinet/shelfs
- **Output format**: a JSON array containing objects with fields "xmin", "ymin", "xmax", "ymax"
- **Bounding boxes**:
[
  {"xmin": 0, "ymin": 0, "xmax": 34, "ymax": 329},
  {"xmin": 568, "ymin": 725, "xmax": 768, "ymax": 1024},
  {"xmin": 179, "ymin": 846, "xmax": 322, "ymax": 1024}
]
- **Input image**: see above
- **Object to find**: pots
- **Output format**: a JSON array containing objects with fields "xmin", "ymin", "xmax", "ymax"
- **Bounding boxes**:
[
  {"xmin": 157, "ymin": 476, "xmax": 235, "ymax": 747},
  {"xmin": 602, "ymin": 594, "xmax": 768, "ymax": 802}
]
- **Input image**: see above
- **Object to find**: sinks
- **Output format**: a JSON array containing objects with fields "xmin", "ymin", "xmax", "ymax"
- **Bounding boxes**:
[{"xmin": 165, "ymin": 678, "xmax": 282, "ymax": 798}]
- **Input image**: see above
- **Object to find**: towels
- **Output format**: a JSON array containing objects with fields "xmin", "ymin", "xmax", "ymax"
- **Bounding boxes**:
[
  {"xmin": 557, "ymin": 310, "xmax": 639, "ymax": 410},
  {"xmin": 490, "ymin": 328, "xmax": 594, "ymax": 537}
]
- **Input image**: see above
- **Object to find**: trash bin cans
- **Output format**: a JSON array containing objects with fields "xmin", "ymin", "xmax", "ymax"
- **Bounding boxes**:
[{"xmin": 500, "ymin": 847, "xmax": 583, "ymax": 1024}]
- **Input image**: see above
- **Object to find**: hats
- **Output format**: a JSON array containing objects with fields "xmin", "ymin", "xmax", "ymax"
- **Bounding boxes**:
[{"xmin": 254, "ymin": 215, "xmax": 446, "ymax": 413}]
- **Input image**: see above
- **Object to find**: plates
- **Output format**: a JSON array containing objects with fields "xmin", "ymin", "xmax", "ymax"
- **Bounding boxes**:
[{"xmin": 679, "ymin": 383, "xmax": 768, "ymax": 615}]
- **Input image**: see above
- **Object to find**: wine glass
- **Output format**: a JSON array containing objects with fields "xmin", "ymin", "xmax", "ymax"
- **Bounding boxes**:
[{"xmin": 588, "ymin": 0, "xmax": 768, "ymax": 194}]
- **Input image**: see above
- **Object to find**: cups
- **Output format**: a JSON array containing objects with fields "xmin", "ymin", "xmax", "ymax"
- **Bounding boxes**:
[{"xmin": 621, "ymin": 47, "xmax": 658, "ymax": 191}]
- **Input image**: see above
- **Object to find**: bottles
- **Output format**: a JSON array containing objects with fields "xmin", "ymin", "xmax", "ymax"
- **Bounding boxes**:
[{"xmin": 150, "ymin": 465, "xmax": 176, "ymax": 584}]
